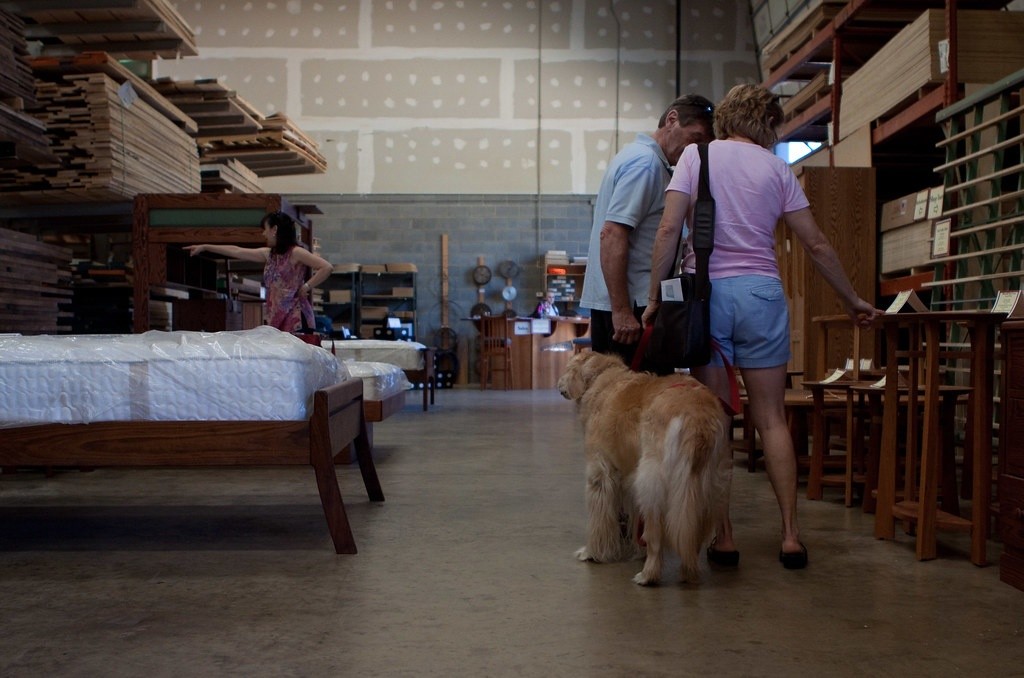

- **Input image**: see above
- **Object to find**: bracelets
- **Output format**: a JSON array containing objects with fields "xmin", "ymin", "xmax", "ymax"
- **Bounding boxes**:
[
  {"xmin": 305, "ymin": 283, "xmax": 311, "ymax": 289},
  {"xmin": 648, "ymin": 297, "xmax": 658, "ymax": 301}
]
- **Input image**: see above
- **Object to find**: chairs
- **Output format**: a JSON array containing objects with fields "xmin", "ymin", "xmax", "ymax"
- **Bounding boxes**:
[
  {"xmin": 479, "ymin": 314, "xmax": 513, "ymax": 391},
  {"xmin": 726, "ymin": 315, "xmax": 976, "ymax": 536},
  {"xmin": 573, "ymin": 339, "xmax": 592, "ymax": 355}
]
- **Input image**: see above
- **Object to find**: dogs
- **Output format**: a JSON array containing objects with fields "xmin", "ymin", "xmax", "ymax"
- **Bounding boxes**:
[{"xmin": 556, "ymin": 350, "xmax": 735, "ymax": 589}]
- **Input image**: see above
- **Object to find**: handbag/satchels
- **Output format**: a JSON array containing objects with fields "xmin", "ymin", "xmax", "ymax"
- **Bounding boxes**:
[
  {"xmin": 640, "ymin": 273, "xmax": 712, "ymax": 375},
  {"xmin": 291, "ymin": 332, "xmax": 322, "ymax": 347}
]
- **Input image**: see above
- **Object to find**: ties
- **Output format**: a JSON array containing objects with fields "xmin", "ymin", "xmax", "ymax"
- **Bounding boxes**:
[{"xmin": 552, "ymin": 304, "xmax": 558, "ymax": 316}]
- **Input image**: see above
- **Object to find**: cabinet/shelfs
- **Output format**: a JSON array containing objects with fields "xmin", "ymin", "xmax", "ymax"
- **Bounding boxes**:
[
  {"xmin": 312, "ymin": 269, "xmax": 419, "ymax": 340},
  {"xmin": 542, "ymin": 261, "xmax": 586, "ymax": 318},
  {"xmin": 171, "ymin": 292, "xmax": 265, "ymax": 334},
  {"xmin": 460, "ymin": 317, "xmax": 593, "ymax": 390},
  {"xmin": 772, "ymin": 164, "xmax": 874, "ymax": 389}
]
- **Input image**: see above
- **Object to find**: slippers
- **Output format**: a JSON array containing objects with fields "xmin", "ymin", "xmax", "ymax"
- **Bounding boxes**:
[
  {"xmin": 707, "ymin": 535, "xmax": 740, "ymax": 568},
  {"xmin": 779, "ymin": 541, "xmax": 810, "ymax": 567}
]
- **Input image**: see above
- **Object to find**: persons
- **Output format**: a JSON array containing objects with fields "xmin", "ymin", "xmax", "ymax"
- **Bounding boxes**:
[
  {"xmin": 579, "ymin": 92, "xmax": 715, "ymax": 540},
  {"xmin": 536, "ymin": 290, "xmax": 559, "ymax": 316},
  {"xmin": 182, "ymin": 211, "xmax": 334, "ymax": 335},
  {"xmin": 640, "ymin": 84, "xmax": 886, "ymax": 569}
]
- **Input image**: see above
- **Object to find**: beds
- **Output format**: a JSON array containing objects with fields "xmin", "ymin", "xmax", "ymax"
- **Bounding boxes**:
[
  {"xmin": 0, "ymin": 330, "xmax": 386, "ymax": 555},
  {"xmin": 320, "ymin": 340, "xmax": 436, "ymax": 412},
  {"xmin": 342, "ymin": 360, "xmax": 412, "ymax": 449}
]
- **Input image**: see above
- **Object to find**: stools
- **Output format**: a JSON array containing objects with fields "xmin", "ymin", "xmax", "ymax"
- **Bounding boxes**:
[{"xmin": 874, "ymin": 308, "xmax": 1024, "ymax": 588}]
